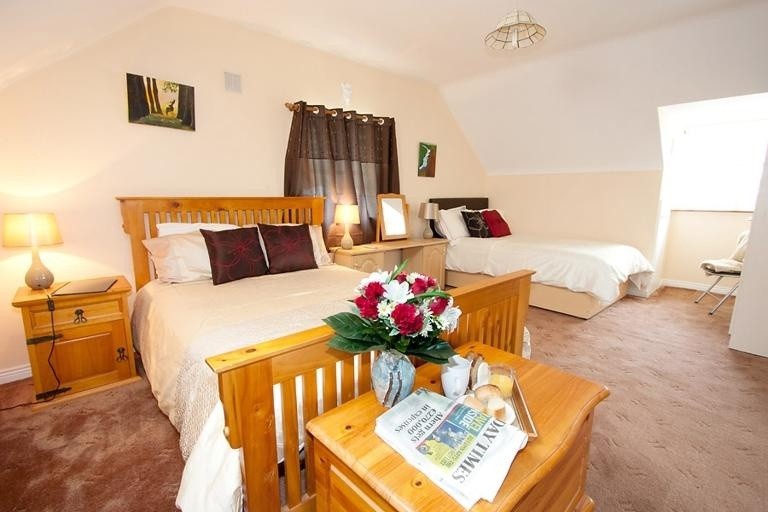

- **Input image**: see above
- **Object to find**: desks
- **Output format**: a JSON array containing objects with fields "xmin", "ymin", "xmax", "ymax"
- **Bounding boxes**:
[
  {"xmin": 308, "ymin": 340, "xmax": 610, "ymax": 511},
  {"xmin": 331, "ymin": 239, "xmax": 451, "ymax": 293}
]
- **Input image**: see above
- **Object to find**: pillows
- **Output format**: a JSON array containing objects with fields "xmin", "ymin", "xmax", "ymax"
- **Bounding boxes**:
[
  {"xmin": 482, "ymin": 208, "xmax": 513, "ymax": 238},
  {"xmin": 139, "ymin": 227, "xmax": 212, "ymax": 287},
  {"xmin": 155, "ymin": 223, "xmax": 243, "ymax": 239},
  {"xmin": 246, "ymin": 221, "xmax": 331, "ymax": 277},
  {"xmin": 257, "ymin": 221, "xmax": 319, "ymax": 275},
  {"xmin": 460, "ymin": 210, "xmax": 492, "ymax": 238},
  {"xmin": 199, "ymin": 224, "xmax": 270, "ymax": 285},
  {"xmin": 438, "ymin": 204, "xmax": 469, "ymax": 242}
]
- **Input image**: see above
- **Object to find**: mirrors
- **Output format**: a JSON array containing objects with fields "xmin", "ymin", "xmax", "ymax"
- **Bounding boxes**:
[{"xmin": 377, "ymin": 192, "xmax": 409, "ymax": 241}]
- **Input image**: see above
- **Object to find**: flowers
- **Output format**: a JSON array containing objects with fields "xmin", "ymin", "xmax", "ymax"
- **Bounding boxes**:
[{"xmin": 324, "ymin": 266, "xmax": 471, "ymax": 364}]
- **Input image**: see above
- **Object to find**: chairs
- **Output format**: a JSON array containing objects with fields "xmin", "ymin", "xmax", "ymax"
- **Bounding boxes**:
[{"xmin": 695, "ymin": 211, "xmax": 753, "ymax": 314}]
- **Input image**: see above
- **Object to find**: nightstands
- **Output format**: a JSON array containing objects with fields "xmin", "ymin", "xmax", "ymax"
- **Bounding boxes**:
[{"xmin": 10, "ymin": 277, "xmax": 142, "ymax": 405}]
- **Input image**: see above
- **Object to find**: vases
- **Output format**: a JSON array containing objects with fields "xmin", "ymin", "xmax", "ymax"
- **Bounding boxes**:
[{"xmin": 366, "ymin": 349, "xmax": 420, "ymax": 409}]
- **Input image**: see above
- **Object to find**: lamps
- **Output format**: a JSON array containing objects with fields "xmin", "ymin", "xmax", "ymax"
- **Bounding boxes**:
[
  {"xmin": 332, "ymin": 197, "xmax": 362, "ymax": 249},
  {"xmin": 416, "ymin": 202, "xmax": 441, "ymax": 239},
  {"xmin": 2, "ymin": 205, "xmax": 66, "ymax": 291},
  {"xmin": 483, "ymin": 2, "xmax": 547, "ymax": 56}
]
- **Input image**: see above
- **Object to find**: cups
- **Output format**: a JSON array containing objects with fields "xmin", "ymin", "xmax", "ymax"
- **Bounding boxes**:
[
  {"xmin": 442, "ymin": 356, "xmax": 471, "ymax": 399},
  {"xmin": 489, "ymin": 366, "xmax": 516, "ymax": 398}
]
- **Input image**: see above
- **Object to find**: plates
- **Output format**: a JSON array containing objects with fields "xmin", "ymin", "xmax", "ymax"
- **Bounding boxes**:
[{"xmin": 456, "ymin": 393, "xmax": 516, "ymax": 426}]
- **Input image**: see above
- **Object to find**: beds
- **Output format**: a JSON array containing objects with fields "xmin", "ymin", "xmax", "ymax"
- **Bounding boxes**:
[
  {"xmin": 115, "ymin": 196, "xmax": 531, "ymax": 510},
  {"xmin": 429, "ymin": 193, "xmax": 656, "ymax": 321}
]
